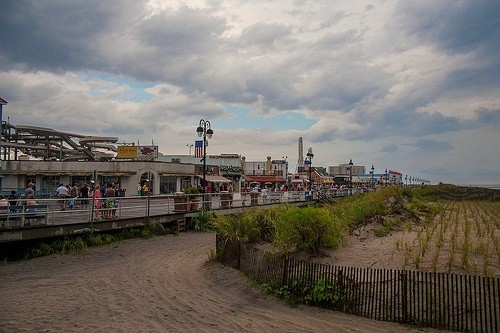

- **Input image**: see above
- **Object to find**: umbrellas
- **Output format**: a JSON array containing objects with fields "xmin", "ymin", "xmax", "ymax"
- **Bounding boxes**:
[{"xmin": 265, "ymin": 182, "xmax": 272, "ymax": 184}]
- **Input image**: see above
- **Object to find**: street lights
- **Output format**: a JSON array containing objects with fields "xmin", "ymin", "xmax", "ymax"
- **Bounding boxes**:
[
  {"xmin": 349, "ymin": 159, "xmax": 354, "ymax": 196},
  {"xmin": 306, "ymin": 152, "xmax": 314, "ymax": 201},
  {"xmin": 369, "ymin": 164, "xmax": 375, "ymax": 188},
  {"xmin": 385, "ymin": 168, "xmax": 387, "ymax": 187},
  {"xmin": 196, "ymin": 118, "xmax": 214, "ymax": 212},
  {"xmin": 399, "ymin": 174, "xmax": 422, "ymax": 186}
]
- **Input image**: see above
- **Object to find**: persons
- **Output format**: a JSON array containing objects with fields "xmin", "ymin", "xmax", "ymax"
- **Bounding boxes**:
[
  {"xmin": 0, "ymin": 182, "xmax": 39, "ymax": 226},
  {"xmin": 94, "ymin": 182, "xmax": 121, "ymax": 221},
  {"xmin": 56, "ymin": 181, "xmax": 91, "ymax": 211},
  {"xmin": 282, "ymin": 184, "xmax": 288, "ymax": 191},
  {"xmin": 137, "ymin": 182, "xmax": 149, "ymax": 196},
  {"xmin": 206, "ymin": 182, "xmax": 235, "ymax": 193}
]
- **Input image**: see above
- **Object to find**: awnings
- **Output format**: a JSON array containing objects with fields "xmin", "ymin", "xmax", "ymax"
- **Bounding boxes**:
[
  {"xmin": 197, "ymin": 175, "xmax": 233, "ymax": 183},
  {"xmin": 292, "ymin": 180, "xmax": 310, "ymax": 183}
]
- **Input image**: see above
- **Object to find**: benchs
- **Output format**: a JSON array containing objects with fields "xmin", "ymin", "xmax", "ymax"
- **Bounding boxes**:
[{"xmin": 0, "ymin": 204, "xmax": 47, "ymax": 225}]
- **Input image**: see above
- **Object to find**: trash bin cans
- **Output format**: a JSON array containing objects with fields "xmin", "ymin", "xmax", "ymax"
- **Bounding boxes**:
[
  {"xmin": 120, "ymin": 188, "xmax": 126, "ymax": 197},
  {"xmin": 173, "ymin": 192, "xmax": 186, "ymax": 209},
  {"xmin": 251, "ymin": 191, "xmax": 259, "ymax": 204},
  {"xmin": 220, "ymin": 191, "xmax": 229, "ymax": 206}
]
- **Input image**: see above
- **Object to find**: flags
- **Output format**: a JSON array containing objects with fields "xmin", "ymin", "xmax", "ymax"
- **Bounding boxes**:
[{"xmin": 195, "ymin": 141, "xmax": 203, "ymax": 158}]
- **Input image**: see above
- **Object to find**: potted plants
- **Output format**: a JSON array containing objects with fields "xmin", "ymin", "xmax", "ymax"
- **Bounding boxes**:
[{"xmin": 182, "ymin": 187, "xmax": 202, "ymax": 211}]
